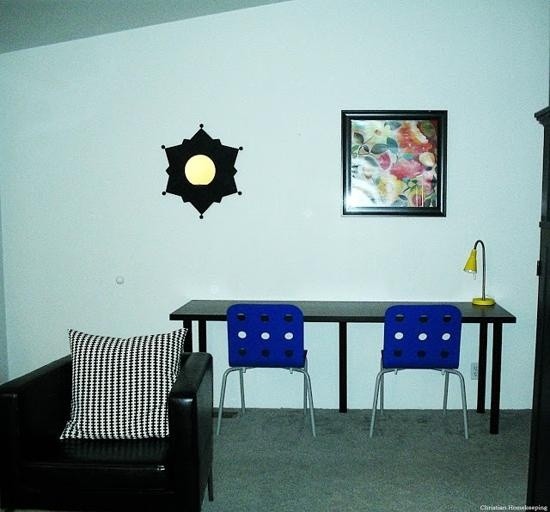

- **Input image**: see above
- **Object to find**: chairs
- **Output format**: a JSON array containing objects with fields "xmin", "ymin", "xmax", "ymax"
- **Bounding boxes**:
[
  {"xmin": 217, "ymin": 303, "xmax": 318, "ymax": 437},
  {"xmin": 366, "ymin": 305, "xmax": 470, "ymax": 440}
]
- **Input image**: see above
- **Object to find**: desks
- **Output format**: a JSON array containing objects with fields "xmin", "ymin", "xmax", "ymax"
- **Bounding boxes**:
[{"xmin": 168, "ymin": 296, "xmax": 518, "ymax": 435}]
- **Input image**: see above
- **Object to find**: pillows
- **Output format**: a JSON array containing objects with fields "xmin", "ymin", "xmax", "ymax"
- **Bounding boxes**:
[{"xmin": 60, "ymin": 326, "xmax": 190, "ymax": 444}]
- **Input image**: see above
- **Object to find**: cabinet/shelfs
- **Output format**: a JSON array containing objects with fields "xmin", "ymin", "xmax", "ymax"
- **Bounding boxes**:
[{"xmin": 524, "ymin": 106, "xmax": 550, "ymax": 511}]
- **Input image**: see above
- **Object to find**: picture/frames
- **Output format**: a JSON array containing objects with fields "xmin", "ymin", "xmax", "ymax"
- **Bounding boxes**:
[{"xmin": 339, "ymin": 108, "xmax": 450, "ymax": 218}]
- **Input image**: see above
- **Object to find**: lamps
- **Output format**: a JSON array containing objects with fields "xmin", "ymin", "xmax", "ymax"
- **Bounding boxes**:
[{"xmin": 464, "ymin": 238, "xmax": 495, "ymax": 307}]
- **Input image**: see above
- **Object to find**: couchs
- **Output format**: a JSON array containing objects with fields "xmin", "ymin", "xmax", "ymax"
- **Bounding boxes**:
[{"xmin": 0, "ymin": 350, "xmax": 217, "ymax": 510}]
